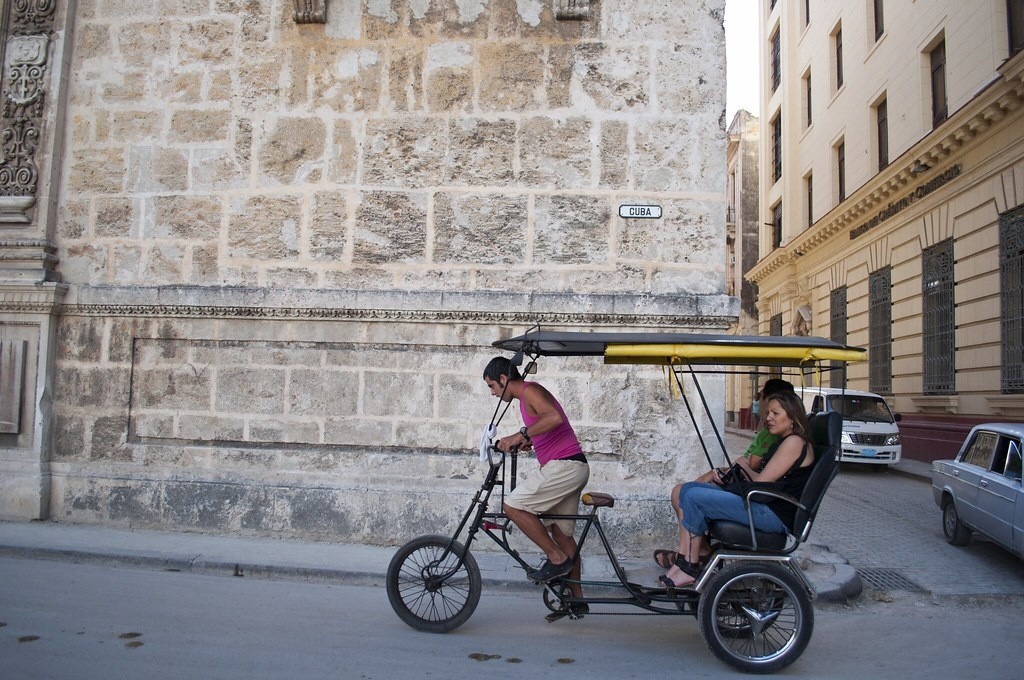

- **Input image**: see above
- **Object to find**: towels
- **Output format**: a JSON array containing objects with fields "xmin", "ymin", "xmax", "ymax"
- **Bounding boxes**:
[{"xmin": 478, "ymin": 423, "xmax": 497, "ymax": 464}]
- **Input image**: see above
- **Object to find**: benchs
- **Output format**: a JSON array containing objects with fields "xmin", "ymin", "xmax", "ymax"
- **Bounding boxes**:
[{"xmin": 705, "ymin": 410, "xmax": 843, "ymax": 554}]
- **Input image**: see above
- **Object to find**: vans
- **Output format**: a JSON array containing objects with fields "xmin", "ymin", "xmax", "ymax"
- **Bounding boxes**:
[{"xmin": 791, "ymin": 385, "xmax": 902, "ymax": 470}]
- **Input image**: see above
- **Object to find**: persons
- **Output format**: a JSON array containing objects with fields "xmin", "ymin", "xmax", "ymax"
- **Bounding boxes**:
[
  {"xmin": 861, "ymin": 400, "xmax": 881, "ymax": 417},
  {"xmin": 819, "ymin": 398, "xmax": 823, "ymax": 412},
  {"xmin": 653, "ymin": 379, "xmax": 814, "ymax": 587},
  {"xmin": 482, "ymin": 356, "xmax": 589, "ymax": 621}
]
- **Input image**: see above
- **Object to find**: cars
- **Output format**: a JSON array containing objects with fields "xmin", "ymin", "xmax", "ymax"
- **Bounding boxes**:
[{"xmin": 931, "ymin": 423, "xmax": 1024, "ymax": 567}]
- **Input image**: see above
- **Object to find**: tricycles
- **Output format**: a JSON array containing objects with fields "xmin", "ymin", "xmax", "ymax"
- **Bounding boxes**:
[{"xmin": 384, "ymin": 320, "xmax": 867, "ymax": 675}]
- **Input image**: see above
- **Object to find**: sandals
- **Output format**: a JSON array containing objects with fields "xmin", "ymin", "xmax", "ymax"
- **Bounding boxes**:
[
  {"xmin": 654, "ymin": 549, "xmax": 679, "ymax": 568},
  {"xmin": 658, "ymin": 561, "xmax": 704, "ymax": 588},
  {"xmin": 657, "ymin": 553, "xmax": 686, "ymax": 583}
]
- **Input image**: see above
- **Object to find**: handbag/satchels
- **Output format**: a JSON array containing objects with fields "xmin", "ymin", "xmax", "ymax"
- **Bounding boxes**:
[{"xmin": 718, "ymin": 463, "xmax": 782, "ymax": 508}]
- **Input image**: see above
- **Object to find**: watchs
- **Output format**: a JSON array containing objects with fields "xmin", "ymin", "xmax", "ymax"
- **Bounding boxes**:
[{"xmin": 520, "ymin": 426, "xmax": 530, "ymax": 442}]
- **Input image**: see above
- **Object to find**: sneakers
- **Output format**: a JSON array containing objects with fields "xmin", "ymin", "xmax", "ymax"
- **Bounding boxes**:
[
  {"xmin": 544, "ymin": 601, "xmax": 589, "ymax": 621},
  {"xmin": 527, "ymin": 557, "xmax": 573, "ymax": 582}
]
